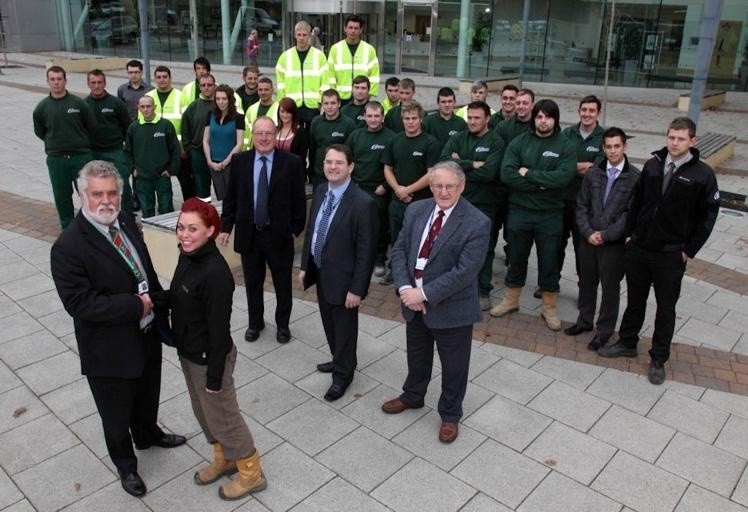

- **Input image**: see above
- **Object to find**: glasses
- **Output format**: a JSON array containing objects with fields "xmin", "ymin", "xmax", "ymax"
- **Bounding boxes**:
[{"xmin": 433, "ymin": 184, "xmax": 457, "ymax": 192}]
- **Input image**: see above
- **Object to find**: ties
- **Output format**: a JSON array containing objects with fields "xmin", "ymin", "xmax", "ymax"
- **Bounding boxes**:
[
  {"xmin": 313, "ymin": 191, "xmax": 334, "ymax": 268},
  {"xmin": 601, "ymin": 168, "xmax": 618, "ymax": 208},
  {"xmin": 413, "ymin": 210, "xmax": 445, "ymax": 279},
  {"xmin": 253, "ymin": 156, "xmax": 269, "ymax": 224},
  {"xmin": 109, "ymin": 226, "xmax": 155, "ymax": 349},
  {"xmin": 662, "ymin": 162, "xmax": 675, "ymax": 194}
]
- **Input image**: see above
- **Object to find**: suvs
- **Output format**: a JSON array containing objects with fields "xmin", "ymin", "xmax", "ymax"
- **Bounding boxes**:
[{"xmin": 88, "ymin": 2, "xmax": 126, "ymax": 14}]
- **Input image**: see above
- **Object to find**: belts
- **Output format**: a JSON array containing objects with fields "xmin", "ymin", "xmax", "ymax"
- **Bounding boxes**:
[{"xmin": 255, "ymin": 224, "xmax": 269, "ymax": 231}]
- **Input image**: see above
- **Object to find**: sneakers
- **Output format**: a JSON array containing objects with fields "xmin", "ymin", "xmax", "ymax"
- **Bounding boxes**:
[
  {"xmin": 375, "ymin": 267, "xmax": 386, "ymax": 276},
  {"xmin": 380, "ymin": 270, "xmax": 392, "ymax": 285},
  {"xmin": 479, "ymin": 290, "xmax": 489, "ymax": 311}
]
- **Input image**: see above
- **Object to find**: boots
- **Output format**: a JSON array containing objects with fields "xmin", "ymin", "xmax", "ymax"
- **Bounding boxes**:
[
  {"xmin": 490, "ymin": 287, "xmax": 525, "ymax": 317},
  {"xmin": 219, "ymin": 448, "xmax": 267, "ymax": 501},
  {"xmin": 541, "ymin": 291, "xmax": 562, "ymax": 330},
  {"xmin": 194, "ymin": 442, "xmax": 239, "ymax": 484}
]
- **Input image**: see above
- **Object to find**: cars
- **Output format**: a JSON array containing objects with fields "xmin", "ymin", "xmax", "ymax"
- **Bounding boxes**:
[
  {"xmin": 88, "ymin": 17, "xmax": 139, "ymax": 46},
  {"xmin": 235, "ymin": 6, "xmax": 280, "ymax": 37}
]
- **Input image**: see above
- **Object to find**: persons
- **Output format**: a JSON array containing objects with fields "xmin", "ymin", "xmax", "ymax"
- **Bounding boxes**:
[
  {"xmin": 297, "ymin": 143, "xmax": 379, "ymax": 401},
  {"xmin": 534, "ymin": 95, "xmax": 605, "ymax": 299},
  {"xmin": 247, "ymin": 27, "xmax": 263, "ymax": 76},
  {"xmin": 117, "ymin": 59, "xmax": 156, "ymax": 212},
  {"xmin": 596, "ymin": 117, "xmax": 720, "ymax": 385},
  {"xmin": 275, "ymin": 20, "xmax": 329, "ymax": 185},
  {"xmin": 346, "ymin": 103, "xmax": 396, "ymax": 276},
  {"xmin": 33, "ymin": 65, "xmax": 96, "ymax": 229},
  {"xmin": 202, "ymin": 85, "xmax": 245, "ymax": 202},
  {"xmin": 310, "ymin": 86, "xmax": 355, "ymax": 194},
  {"xmin": 440, "ymin": 102, "xmax": 505, "ymax": 311},
  {"xmin": 50, "ymin": 159, "xmax": 187, "ymax": 496},
  {"xmin": 124, "ymin": 97, "xmax": 182, "ymax": 219},
  {"xmin": 380, "ymin": 161, "xmax": 491, "ymax": 442},
  {"xmin": 181, "ymin": 56, "xmax": 211, "ymax": 112},
  {"xmin": 244, "ymin": 78, "xmax": 279, "ymax": 152},
  {"xmin": 342, "ymin": 74, "xmax": 372, "ymax": 128},
  {"xmin": 383, "ymin": 78, "xmax": 428, "ymax": 134},
  {"xmin": 169, "ymin": 197, "xmax": 267, "ymax": 500},
  {"xmin": 486, "ymin": 84, "xmax": 519, "ymax": 130},
  {"xmin": 490, "ymin": 99, "xmax": 578, "ymax": 331},
  {"xmin": 455, "ymin": 80, "xmax": 495, "ymax": 124},
  {"xmin": 565, "ymin": 127, "xmax": 640, "ymax": 350},
  {"xmin": 329, "ymin": 14, "xmax": 380, "ymax": 109},
  {"xmin": 423, "ymin": 87, "xmax": 467, "ymax": 145},
  {"xmin": 381, "ymin": 76, "xmax": 400, "ymax": 117},
  {"xmin": 493, "ymin": 90, "xmax": 535, "ymax": 143},
  {"xmin": 82, "ymin": 69, "xmax": 135, "ymax": 213},
  {"xmin": 275, "ymin": 98, "xmax": 307, "ymax": 159},
  {"xmin": 220, "ymin": 116, "xmax": 308, "ymax": 343},
  {"xmin": 381, "ymin": 101, "xmax": 440, "ymax": 285},
  {"xmin": 237, "ymin": 68, "xmax": 260, "ymax": 112},
  {"xmin": 137, "ymin": 65, "xmax": 195, "ymax": 201},
  {"xmin": 182, "ymin": 73, "xmax": 216, "ymax": 204}
]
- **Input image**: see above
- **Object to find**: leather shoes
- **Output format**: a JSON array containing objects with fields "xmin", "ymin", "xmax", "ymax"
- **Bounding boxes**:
[
  {"xmin": 439, "ymin": 420, "xmax": 458, "ymax": 443},
  {"xmin": 135, "ymin": 434, "xmax": 186, "ymax": 449},
  {"xmin": 650, "ymin": 359, "xmax": 666, "ymax": 385},
  {"xmin": 317, "ymin": 361, "xmax": 337, "ymax": 373},
  {"xmin": 324, "ymin": 377, "xmax": 353, "ymax": 401},
  {"xmin": 588, "ymin": 335, "xmax": 607, "ymax": 351},
  {"xmin": 382, "ymin": 396, "xmax": 408, "ymax": 413},
  {"xmin": 117, "ymin": 465, "xmax": 146, "ymax": 496},
  {"xmin": 598, "ymin": 341, "xmax": 637, "ymax": 357},
  {"xmin": 277, "ymin": 327, "xmax": 290, "ymax": 343},
  {"xmin": 565, "ymin": 325, "xmax": 591, "ymax": 335},
  {"xmin": 245, "ymin": 322, "xmax": 265, "ymax": 341}
]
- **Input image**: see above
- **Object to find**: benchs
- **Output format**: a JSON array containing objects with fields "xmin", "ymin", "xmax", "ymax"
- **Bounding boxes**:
[
  {"xmin": 692, "ymin": 131, "xmax": 736, "ymax": 170},
  {"xmin": 644, "ymin": 72, "xmax": 738, "ymax": 89},
  {"xmin": 678, "ymin": 89, "xmax": 726, "ymax": 112},
  {"xmin": 563, "ymin": 69, "xmax": 616, "ymax": 80},
  {"xmin": 141, "ymin": 183, "xmax": 313, "ymax": 283},
  {"xmin": 459, "ymin": 75, "xmax": 519, "ymax": 92},
  {"xmin": 499, "ymin": 66, "xmax": 551, "ymax": 79},
  {"xmin": 47, "ymin": 58, "xmax": 131, "ymax": 73}
]
